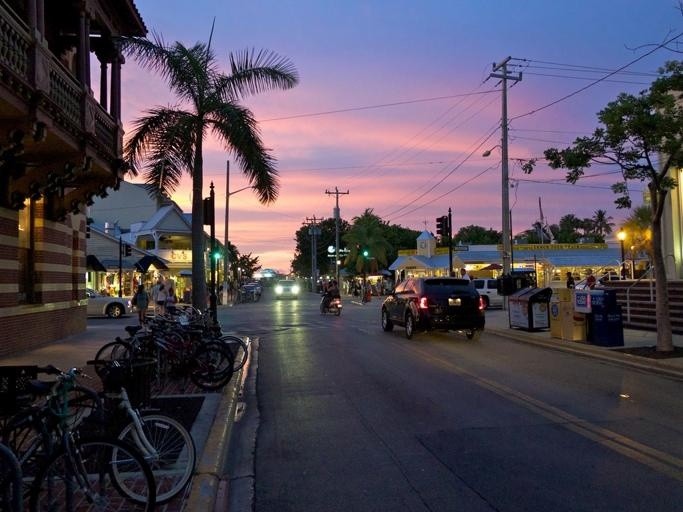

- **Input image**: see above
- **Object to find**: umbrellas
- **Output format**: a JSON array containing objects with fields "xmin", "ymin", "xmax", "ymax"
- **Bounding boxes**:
[{"xmin": 478, "ymin": 262, "xmax": 502, "ymax": 271}]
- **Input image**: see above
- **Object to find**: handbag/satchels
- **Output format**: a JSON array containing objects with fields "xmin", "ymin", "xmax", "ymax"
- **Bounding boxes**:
[{"xmin": 131, "ymin": 296, "xmax": 138, "ymax": 306}]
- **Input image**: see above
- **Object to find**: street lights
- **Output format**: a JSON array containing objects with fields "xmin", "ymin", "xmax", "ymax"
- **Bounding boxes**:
[
  {"xmin": 618, "ymin": 227, "xmax": 626, "ymax": 280},
  {"xmin": 481, "ymin": 141, "xmax": 513, "ymax": 273}
]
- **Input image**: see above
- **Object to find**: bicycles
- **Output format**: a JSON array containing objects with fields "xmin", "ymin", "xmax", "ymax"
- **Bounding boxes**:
[{"xmin": 1, "ymin": 305, "xmax": 248, "ymax": 512}]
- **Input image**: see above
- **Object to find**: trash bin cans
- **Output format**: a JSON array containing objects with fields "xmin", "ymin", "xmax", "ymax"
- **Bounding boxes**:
[{"xmin": 508, "ymin": 285, "xmax": 552, "ymax": 332}]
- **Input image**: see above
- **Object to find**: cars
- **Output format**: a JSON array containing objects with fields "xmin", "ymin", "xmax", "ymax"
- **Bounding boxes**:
[
  {"xmin": 83, "ymin": 288, "xmax": 133, "ymax": 318},
  {"xmin": 242, "ymin": 268, "xmax": 301, "ymax": 301}
]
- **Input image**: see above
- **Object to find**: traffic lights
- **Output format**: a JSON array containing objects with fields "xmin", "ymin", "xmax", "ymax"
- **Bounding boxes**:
[
  {"xmin": 435, "ymin": 215, "xmax": 448, "ymax": 237},
  {"xmin": 363, "ymin": 250, "xmax": 368, "ymax": 259},
  {"xmin": 214, "ymin": 250, "xmax": 221, "ymax": 261}
]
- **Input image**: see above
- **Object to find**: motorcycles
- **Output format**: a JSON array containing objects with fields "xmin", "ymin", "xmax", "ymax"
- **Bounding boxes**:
[{"xmin": 318, "ymin": 292, "xmax": 342, "ymax": 316}]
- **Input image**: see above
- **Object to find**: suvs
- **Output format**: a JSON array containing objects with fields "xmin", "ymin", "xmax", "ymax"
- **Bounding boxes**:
[{"xmin": 379, "ymin": 275, "xmax": 486, "ymax": 340}]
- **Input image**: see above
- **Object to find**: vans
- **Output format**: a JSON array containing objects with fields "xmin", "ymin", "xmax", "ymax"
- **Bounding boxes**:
[{"xmin": 468, "ymin": 277, "xmax": 505, "ymax": 309}]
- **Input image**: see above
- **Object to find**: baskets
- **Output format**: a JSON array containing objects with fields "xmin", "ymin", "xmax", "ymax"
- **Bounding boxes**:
[{"xmin": 102, "ymin": 357, "xmax": 157, "ymax": 406}]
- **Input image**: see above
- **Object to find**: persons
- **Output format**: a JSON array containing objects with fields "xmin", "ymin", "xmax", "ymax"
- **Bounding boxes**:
[
  {"xmin": 134, "ymin": 280, "xmax": 168, "ymax": 325},
  {"xmin": 459, "ymin": 268, "xmax": 469, "ymax": 280},
  {"xmin": 583, "ymin": 269, "xmax": 595, "ymax": 290},
  {"xmin": 318, "ymin": 278, "xmax": 341, "ymax": 315},
  {"xmin": 163, "ymin": 287, "xmax": 178, "ymax": 321},
  {"xmin": 566, "ymin": 271, "xmax": 575, "ymax": 289},
  {"xmin": 343, "ymin": 278, "xmax": 384, "ymax": 305}
]
